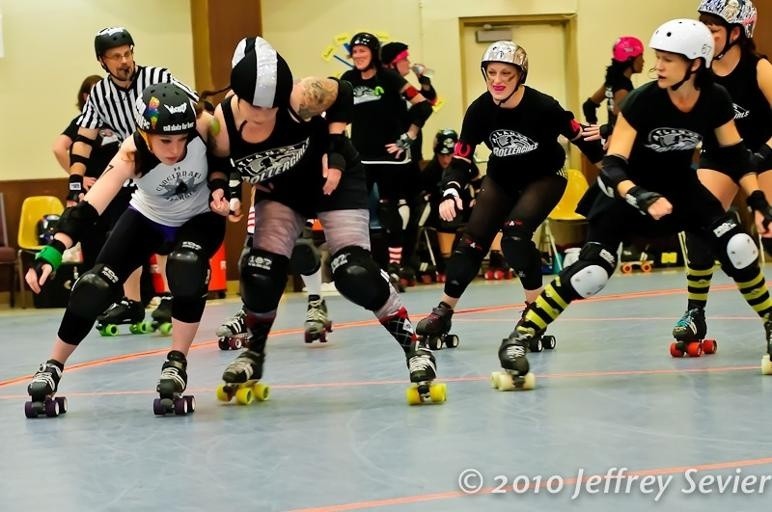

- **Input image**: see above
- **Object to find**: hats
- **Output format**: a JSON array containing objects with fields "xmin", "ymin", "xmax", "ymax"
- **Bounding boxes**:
[{"xmin": 349, "ymin": 32, "xmax": 381, "ymax": 68}]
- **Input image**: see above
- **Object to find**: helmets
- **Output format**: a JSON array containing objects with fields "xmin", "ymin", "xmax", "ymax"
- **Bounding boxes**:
[
  {"xmin": 649, "ymin": 17, "xmax": 716, "ymax": 70},
  {"xmin": 132, "ymin": 82, "xmax": 197, "ymax": 134},
  {"xmin": 480, "ymin": 40, "xmax": 530, "ymax": 83},
  {"xmin": 696, "ymin": 0, "xmax": 758, "ymax": 39},
  {"xmin": 611, "ymin": 36, "xmax": 644, "ymax": 64},
  {"xmin": 94, "ymin": 25, "xmax": 135, "ymax": 61},
  {"xmin": 229, "ymin": 36, "xmax": 295, "ymax": 108},
  {"xmin": 36, "ymin": 213, "xmax": 64, "ymax": 246},
  {"xmin": 383, "ymin": 40, "xmax": 409, "ymax": 67},
  {"xmin": 432, "ymin": 128, "xmax": 460, "ymax": 156}
]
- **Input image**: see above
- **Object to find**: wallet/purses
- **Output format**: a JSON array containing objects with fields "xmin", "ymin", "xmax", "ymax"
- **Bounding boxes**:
[{"xmin": 322, "ymin": 133, "xmax": 350, "ymax": 172}]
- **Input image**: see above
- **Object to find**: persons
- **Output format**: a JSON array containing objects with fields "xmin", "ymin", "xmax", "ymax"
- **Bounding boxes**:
[{"xmin": 27, "ymin": 2, "xmax": 772, "ymax": 399}]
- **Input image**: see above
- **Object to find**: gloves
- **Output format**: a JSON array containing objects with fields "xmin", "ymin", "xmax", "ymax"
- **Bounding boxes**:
[
  {"xmin": 205, "ymin": 177, "xmax": 246, "ymax": 224},
  {"xmin": 745, "ymin": 188, "xmax": 772, "ymax": 230},
  {"xmin": 438, "ymin": 181, "xmax": 463, "ymax": 203},
  {"xmin": 626, "ymin": 184, "xmax": 666, "ymax": 217},
  {"xmin": 30, "ymin": 238, "xmax": 66, "ymax": 280}
]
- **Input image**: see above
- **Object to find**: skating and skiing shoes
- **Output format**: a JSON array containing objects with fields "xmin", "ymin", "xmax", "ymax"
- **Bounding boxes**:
[
  {"xmin": 416, "ymin": 304, "xmax": 460, "ymax": 350},
  {"xmin": 482, "ymin": 251, "xmax": 513, "ymax": 281},
  {"xmin": 667, "ymin": 308, "xmax": 718, "ymax": 359},
  {"xmin": 153, "ymin": 350, "xmax": 197, "ymax": 417},
  {"xmin": 304, "ymin": 294, "xmax": 334, "ymax": 346},
  {"xmin": 619, "ymin": 245, "xmax": 657, "ymax": 273},
  {"xmin": 217, "ymin": 349, "xmax": 270, "ymax": 405},
  {"xmin": 24, "ymin": 358, "xmax": 67, "ymax": 417},
  {"xmin": 405, "ymin": 350, "xmax": 448, "ymax": 406},
  {"xmin": 214, "ymin": 309, "xmax": 251, "ymax": 350},
  {"xmin": 488, "ymin": 339, "xmax": 536, "ymax": 390},
  {"xmin": 93, "ymin": 292, "xmax": 174, "ymax": 336},
  {"xmin": 387, "ymin": 256, "xmax": 447, "ymax": 292}
]
